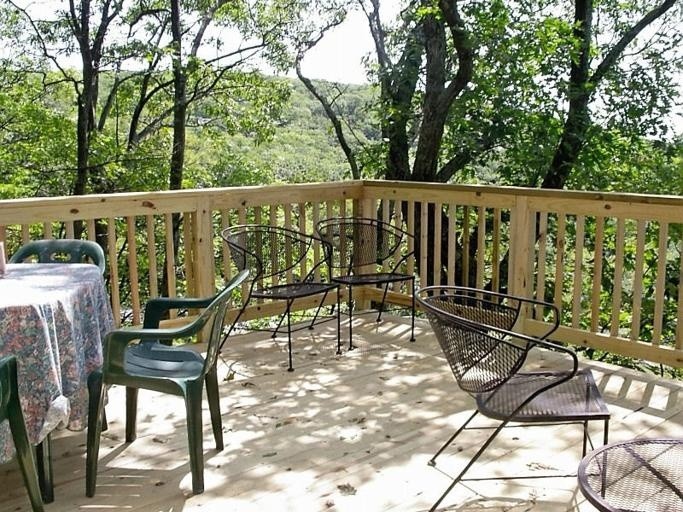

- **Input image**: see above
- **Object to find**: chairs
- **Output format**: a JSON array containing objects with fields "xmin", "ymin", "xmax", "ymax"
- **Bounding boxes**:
[
  {"xmin": 415, "ymin": 285, "xmax": 612, "ymax": 512},
  {"xmin": 220, "ymin": 216, "xmax": 417, "ymax": 372},
  {"xmin": 0, "ymin": 354, "xmax": 47, "ymax": 512},
  {"xmin": 10, "ymin": 239, "xmax": 107, "ymax": 275},
  {"xmin": 85, "ymin": 268, "xmax": 253, "ymax": 496}
]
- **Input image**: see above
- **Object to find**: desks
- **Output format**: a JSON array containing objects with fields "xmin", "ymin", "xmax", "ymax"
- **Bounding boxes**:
[
  {"xmin": 578, "ymin": 439, "xmax": 683, "ymax": 512},
  {"xmin": 0, "ymin": 262, "xmax": 107, "ymax": 504}
]
